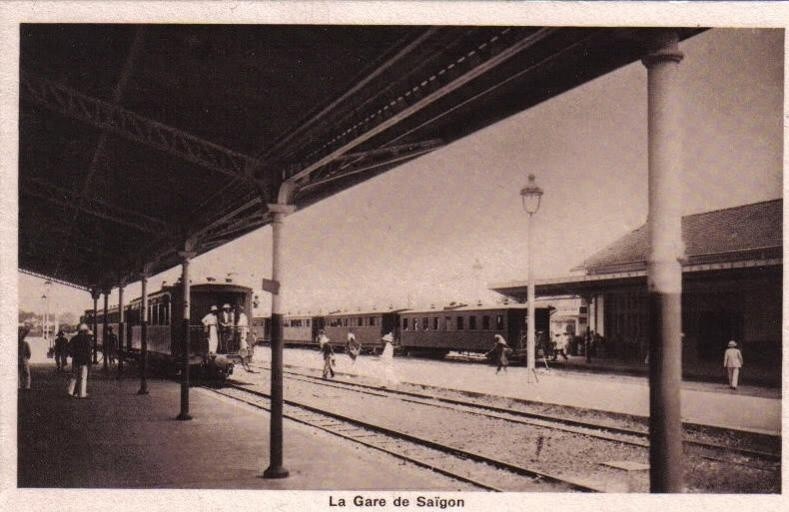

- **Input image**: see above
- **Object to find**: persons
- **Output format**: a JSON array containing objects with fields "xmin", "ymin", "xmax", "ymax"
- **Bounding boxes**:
[
  {"xmin": 379, "ymin": 332, "xmax": 395, "ymax": 360},
  {"xmin": 63, "ymin": 324, "xmax": 95, "ymax": 398},
  {"xmin": 200, "ymin": 303, "xmax": 250, "ymax": 356},
  {"xmin": 317, "ymin": 328, "xmax": 328, "ymax": 353},
  {"xmin": 319, "ymin": 342, "xmax": 335, "ymax": 379},
  {"xmin": 343, "ymin": 335, "xmax": 361, "ymax": 364},
  {"xmin": 532, "ymin": 327, "xmax": 605, "ymax": 362},
  {"xmin": 347, "ymin": 327, "xmax": 355, "ymax": 342},
  {"xmin": 104, "ymin": 325, "xmax": 120, "ymax": 370},
  {"xmin": 51, "ymin": 329, "xmax": 70, "ymax": 371},
  {"xmin": 721, "ymin": 340, "xmax": 746, "ymax": 390},
  {"xmin": 484, "ymin": 333, "xmax": 513, "ymax": 375},
  {"xmin": 18, "ymin": 325, "xmax": 33, "ymax": 394}
]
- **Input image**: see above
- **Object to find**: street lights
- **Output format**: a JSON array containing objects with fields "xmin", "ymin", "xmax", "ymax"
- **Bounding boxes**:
[
  {"xmin": 519, "ymin": 174, "xmax": 544, "ymax": 383},
  {"xmin": 41, "ymin": 281, "xmax": 53, "ymax": 344}
]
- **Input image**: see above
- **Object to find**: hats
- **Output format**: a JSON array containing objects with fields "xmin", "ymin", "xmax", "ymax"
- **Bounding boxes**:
[
  {"xmin": 210, "ymin": 303, "xmax": 231, "ymax": 311},
  {"xmin": 79, "ymin": 323, "xmax": 88, "ymax": 330},
  {"xmin": 728, "ymin": 340, "xmax": 738, "ymax": 347}
]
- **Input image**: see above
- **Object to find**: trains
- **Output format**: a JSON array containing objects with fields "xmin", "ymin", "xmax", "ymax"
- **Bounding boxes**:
[
  {"xmin": 79, "ymin": 281, "xmax": 261, "ymax": 379},
  {"xmin": 252, "ymin": 307, "xmax": 550, "ymax": 365}
]
